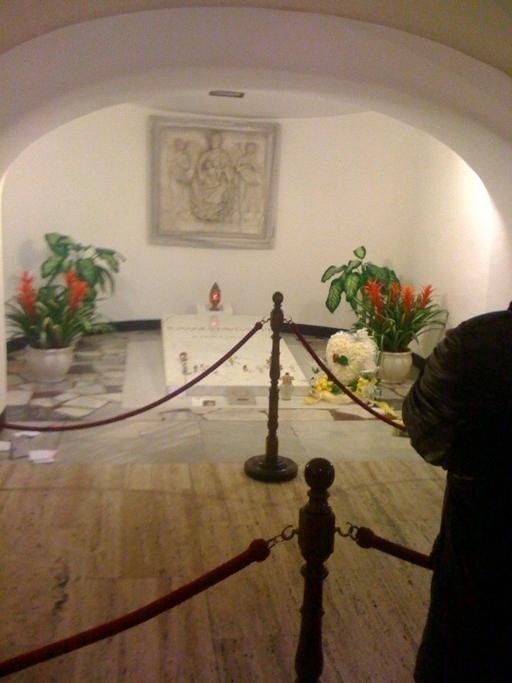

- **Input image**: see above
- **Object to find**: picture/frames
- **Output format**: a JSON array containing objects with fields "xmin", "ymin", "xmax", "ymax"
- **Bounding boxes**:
[{"xmin": 146, "ymin": 113, "xmax": 280, "ymax": 251}]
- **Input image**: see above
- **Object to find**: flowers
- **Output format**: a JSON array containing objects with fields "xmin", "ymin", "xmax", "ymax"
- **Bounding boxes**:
[
  {"xmin": 4, "ymin": 267, "xmax": 117, "ymax": 356},
  {"xmin": 347, "ymin": 278, "xmax": 450, "ymax": 357}
]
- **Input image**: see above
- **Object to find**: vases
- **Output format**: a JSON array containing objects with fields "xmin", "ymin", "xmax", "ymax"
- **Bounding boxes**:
[
  {"xmin": 378, "ymin": 350, "xmax": 413, "ymax": 384},
  {"xmin": 25, "ymin": 344, "xmax": 75, "ymax": 384}
]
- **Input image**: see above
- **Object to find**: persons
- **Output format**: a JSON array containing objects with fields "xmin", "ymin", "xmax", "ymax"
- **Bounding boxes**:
[
  {"xmin": 166, "ymin": 136, "xmax": 193, "ymax": 191},
  {"xmin": 402, "ymin": 295, "xmax": 512, "ymax": 683},
  {"xmin": 189, "ymin": 130, "xmax": 236, "ymax": 220},
  {"xmin": 233, "ymin": 140, "xmax": 264, "ymax": 209}
]
